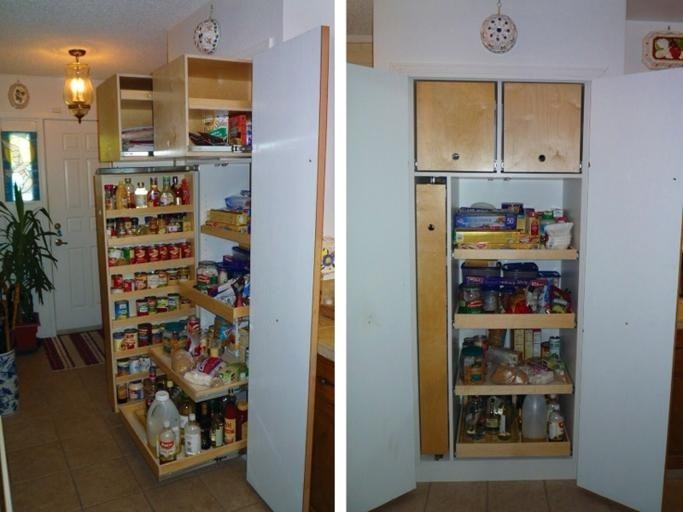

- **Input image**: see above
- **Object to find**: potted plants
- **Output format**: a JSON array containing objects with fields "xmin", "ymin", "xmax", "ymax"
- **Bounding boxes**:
[
  {"xmin": 1, "ymin": 183, "xmax": 58, "ymax": 418},
  {"xmin": 0, "ymin": 280, "xmax": 58, "ymax": 355}
]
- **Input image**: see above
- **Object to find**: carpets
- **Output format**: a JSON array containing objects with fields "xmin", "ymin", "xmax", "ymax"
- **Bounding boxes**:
[{"xmin": 45, "ymin": 329, "xmax": 106, "ymax": 372}]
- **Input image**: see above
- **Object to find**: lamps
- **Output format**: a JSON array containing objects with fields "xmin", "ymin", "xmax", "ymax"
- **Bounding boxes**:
[{"xmin": 63, "ymin": 49, "xmax": 95, "ymax": 125}]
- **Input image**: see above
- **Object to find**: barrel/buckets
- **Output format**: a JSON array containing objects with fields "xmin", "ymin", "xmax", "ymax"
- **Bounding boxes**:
[
  {"xmin": 520, "ymin": 393, "xmax": 549, "ymax": 442},
  {"xmin": 147, "ymin": 390, "xmax": 182, "ymax": 459}
]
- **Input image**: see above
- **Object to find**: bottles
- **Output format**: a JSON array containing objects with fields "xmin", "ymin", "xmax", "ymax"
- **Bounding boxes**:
[
  {"xmin": 104, "ymin": 176, "xmax": 191, "ymax": 238},
  {"xmin": 196, "ymin": 261, "xmax": 229, "ymax": 294},
  {"xmin": 460, "ymin": 346, "xmax": 485, "ymax": 384},
  {"xmin": 145, "ymin": 367, "xmax": 248, "ymax": 465},
  {"xmin": 457, "ymin": 282, "xmax": 531, "ymax": 315},
  {"xmin": 527, "ymin": 211, "xmax": 553, "ymax": 249},
  {"xmin": 483, "ymin": 395, "xmax": 566, "ymax": 442}
]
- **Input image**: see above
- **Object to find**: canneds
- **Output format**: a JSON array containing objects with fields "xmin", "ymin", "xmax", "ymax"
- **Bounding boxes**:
[
  {"xmin": 110, "ymin": 241, "xmax": 192, "ymax": 352},
  {"xmin": 549, "ymin": 336, "xmax": 561, "ymax": 355}
]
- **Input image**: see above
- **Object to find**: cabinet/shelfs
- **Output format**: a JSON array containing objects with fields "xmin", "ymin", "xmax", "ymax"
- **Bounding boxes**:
[
  {"xmin": 308, "ymin": 352, "xmax": 334, "ymax": 512},
  {"xmin": 414, "ymin": 174, "xmax": 447, "ymax": 455},
  {"xmin": 454, "ymin": 193, "xmax": 577, "ymax": 455},
  {"xmin": 119, "ymin": 224, "xmax": 250, "ymax": 481},
  {"xmin": 152, "ymin": 53, "xmax": 253, "ymax": 159},
  {"xmin": 94, "ymin": 74, "xmax": 156, "ymax": 160},
  {"xmin": 414, "ymin": 80, "xmax": 582, "ymax": 176},
  {"xmin": 93, "ymin": 163, "xmax": 197, "ymax": 414}
]
